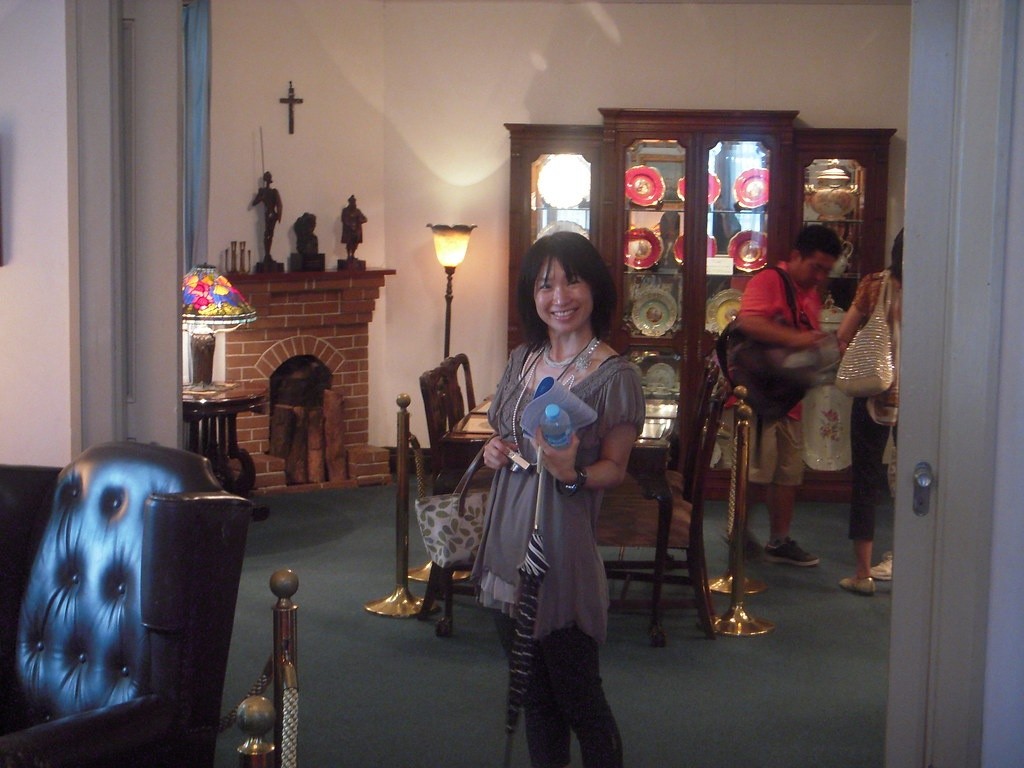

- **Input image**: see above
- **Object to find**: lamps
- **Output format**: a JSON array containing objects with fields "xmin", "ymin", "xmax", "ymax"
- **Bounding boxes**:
[
  {"xmin": 427, "ymin": 224, "xmax": 479, "ymax": 358},
  {"xmin": 182, "ymin": 263, "xmax": 257, "ymax": 390}
]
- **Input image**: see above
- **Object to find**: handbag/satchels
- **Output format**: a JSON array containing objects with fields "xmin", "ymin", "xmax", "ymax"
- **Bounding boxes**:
[
  {"xmin": 786, "ymin": 333, "xmax": 842, "ymax": 378},
  {"xmin": 834, "ymin": 269, "xmax": 893, "ymax": 398},
  {"xmin": 714, "ymin": 265, "xmax": 815, "ymax": 423},
  {"xmin": 415, "ymin": 432, "xmax": 502, "ymax": 570}
]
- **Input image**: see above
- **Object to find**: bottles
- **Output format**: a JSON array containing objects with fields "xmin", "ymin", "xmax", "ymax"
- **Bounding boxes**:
[
  {"xmin": 807, "ymin": 169, "xmax": 859, "ymax": 221},
  {"xmin": 539, "ymin": 404, "xmax": 572, "ymax": 449},
  {"xmin": 817, "ymin": 298, "xmax": 844, "ymax": 332}
]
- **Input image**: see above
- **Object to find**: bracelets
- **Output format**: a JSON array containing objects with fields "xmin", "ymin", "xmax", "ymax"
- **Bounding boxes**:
[{"xmin": 554, "ymin": 466, "xmax": 585, "ymax": 498}]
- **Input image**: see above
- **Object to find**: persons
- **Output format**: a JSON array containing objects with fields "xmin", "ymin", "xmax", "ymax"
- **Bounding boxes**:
[
  {"xmin": 835, "ymin": 228, "xmax": 904, "ymax": 594},
  {"xmin": 339, "ymin": 195, "xmax": 368, "ymax": 261},
  {"xmin": 253, "ymin": 171, "xmax": 283, "ymax": 259},
  {"xmin": 723, "ymin": 224, "xmax": 841, "ymax": 567},
  {"xmin": 650, "ymin": 210, "xmax": 743, "ymax": 303},
  {"xmin": 472, "ymin": 231, "xmax": 646, "ymax": 768}
]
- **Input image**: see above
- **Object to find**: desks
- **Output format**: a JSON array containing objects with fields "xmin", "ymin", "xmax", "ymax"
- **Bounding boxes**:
[
  {"xmin": 183, "ymin": 392, "xmax": 269, "ymax": 519},
  {"xmin": 433, "ymin": 399, "xmax": 678, "ymax": 646}
]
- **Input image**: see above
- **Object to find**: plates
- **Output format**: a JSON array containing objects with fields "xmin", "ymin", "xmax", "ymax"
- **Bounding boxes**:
[
  {"xmin": 797, "ymin": 390, "xmax": 856, "ymax": 471},
  {"xmin": 536, "ymin": 221, "xmax": 588, "ymax": 243},
  {"xmin": 538, "ymin": 155, "xmax": 591, "ymax": 210},
  {"xmin": 627, "ymin": 165, "xmax": 769, "ymax": 394}
]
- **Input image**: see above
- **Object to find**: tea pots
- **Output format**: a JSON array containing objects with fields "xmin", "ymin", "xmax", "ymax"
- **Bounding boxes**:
[{"xmin": 828, "ymin": 240, "xmax": 854, "ymax": 276}]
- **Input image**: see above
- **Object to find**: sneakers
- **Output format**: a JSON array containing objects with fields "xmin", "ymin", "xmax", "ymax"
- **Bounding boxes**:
[
  {"xmin": 870, "ymin": 557, "xmax": 892, "ymax": 580},
  {"xmin": 839, "ymin": 576, "xmax": 875, "ymax": 596},
  {"xmin": 763, "ymin": 536, "xmax": 820, "ymax": 566}
]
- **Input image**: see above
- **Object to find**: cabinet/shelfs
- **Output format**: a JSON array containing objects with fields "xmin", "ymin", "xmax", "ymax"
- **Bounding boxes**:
[{"xmin": 502, "ymin": 108, "xmax": 896, "ymax": 501}]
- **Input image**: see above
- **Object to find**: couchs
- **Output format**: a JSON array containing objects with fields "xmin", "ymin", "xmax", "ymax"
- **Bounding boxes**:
[{"xmin": 0, "ymin": 438, "xmax": 250, "ymax": 768}]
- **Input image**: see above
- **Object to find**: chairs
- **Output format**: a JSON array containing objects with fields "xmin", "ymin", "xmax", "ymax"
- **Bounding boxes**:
[
  {"xmin": 418, "ymin": 354, "xmax": 474, "ymax": 617},
  {"xmin": 603, "ymin": 359, "xmax": 730, "ymax": 640}
]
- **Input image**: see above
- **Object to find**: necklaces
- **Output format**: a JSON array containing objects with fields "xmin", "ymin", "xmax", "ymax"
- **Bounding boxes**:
[{"xmin": 495, "ymin": 337, "xmax": 600, "ymax": 464}]
